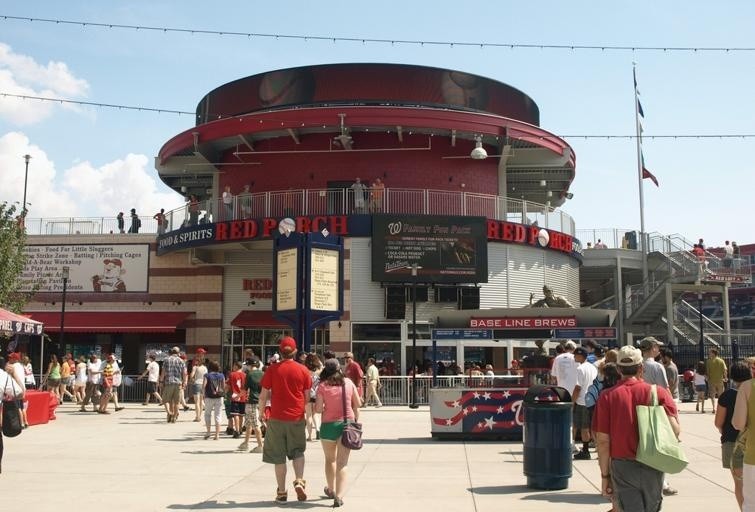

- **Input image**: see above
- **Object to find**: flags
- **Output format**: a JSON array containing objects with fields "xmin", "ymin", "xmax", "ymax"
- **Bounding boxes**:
[{"xmin": 633, "ymin": 71, "xmax": 660, "ymax": 188}]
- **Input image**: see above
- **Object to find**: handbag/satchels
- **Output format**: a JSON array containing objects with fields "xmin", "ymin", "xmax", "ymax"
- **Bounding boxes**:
[
  {"xmin": 1, "ymin": 398, "xmax": 22, "ymax": 437},
  {"xmin": 341, "ymin": 422, "xmax": 363, "ymax": 449},
  {"xmin": 635, "ymin": 384, "xmax": 688, "ymax": 474},
  {"xmin": 729, "ymin": 426, "xmax": 748, "ymax": 480}
]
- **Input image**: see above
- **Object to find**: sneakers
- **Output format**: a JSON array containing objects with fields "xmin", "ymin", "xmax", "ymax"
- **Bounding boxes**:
[
  {"xmin": 573, "ymin": 451, "xmax": 590, "ymax": 460},
  {"xmin": 663, "ymin": 484, "xmax": 677, "ymax": 495},
  {"xmin": 204, "ymin": 427, "xmax": 264, "ymax": 452},
  {"xmin": 276, "ymin": 478, "xmax": 344, "ymax": 507}
]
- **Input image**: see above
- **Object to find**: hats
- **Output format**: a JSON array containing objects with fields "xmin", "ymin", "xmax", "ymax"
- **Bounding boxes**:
[
  {"xmin": 279, "ymin": 337, "xmax": 296, "ymax": 354},
  {"xmin": 639, "ymin": 336, "xmax": 663, "ymax": 351},
  {"xmin": 617, "ymin": 345, "xmax": 643, "ymax": 366},
  {"xmin": 325, "ymin": 358, "xmax": 340, "ymax": 374},
  {"xmin": 572, "ymin": 347, "xmax": 588, "ymax": 357}
]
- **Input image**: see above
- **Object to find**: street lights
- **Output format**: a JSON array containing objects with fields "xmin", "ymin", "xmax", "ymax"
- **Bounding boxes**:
[
  {"xmin": 22, "ymin": 154, "xmax": 32, "ymax": 229},
  {"xmin": 696, "ymin": 289, "xmax": 707, "ymax": 362},
  {"xmin": 407, "ymin": 262, "xmax": 425, "ymax": 409},
  {"xmin": 58, "ymin": 265, "xmax": 69, "ymax": 366}
]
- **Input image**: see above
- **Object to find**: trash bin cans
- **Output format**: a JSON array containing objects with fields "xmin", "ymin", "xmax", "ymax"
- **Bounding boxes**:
[
  {"xmin": 522, "ymin": 383, "xmax": 574, "ymax": 490},
  {"xmin": 678, "ymin": 376, "xmax": 692, "ymax": 403}
]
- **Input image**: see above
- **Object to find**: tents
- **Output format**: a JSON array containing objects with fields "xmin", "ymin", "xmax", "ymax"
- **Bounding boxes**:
[{"xmin": 0, "ymin": 307, "xmax": 44, "ymax": 392}]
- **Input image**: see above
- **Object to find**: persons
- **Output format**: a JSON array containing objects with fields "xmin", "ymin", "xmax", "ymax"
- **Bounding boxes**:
[
  {"xmin": 80, "ymin": 353, "xmax": 123, "ymax": 414},
  {"xmin": 223, "ymin": 185, "xmax": 252, "ymax": 220},
  {"xmin": 45, "ymin": 353, "xmax": 87, "ymax": 405},
  {"xmin": 587, "ymin": 239, "xmax": 603, "ymax": 248},
  {"xmin": 260, "ymin": 336, "xmax": 312, "ymax": 504},
  {"xmin": 224, "ymin": 349, "xmax": 335, "ymax": 452},
  {"xmin": 438, "ymin": 361, "xmax": 494, "ymax": 388},
  {"xmin": 187, "ymin": 194, "xmax": 211, "ymax": 226},
  {"xmin": 160, "ymin": 347, "xmax": 208, "ymax": 422},
  {"xmin": 6, "ymin": 353, "xmax": 36, "ymax": 429},
  {"xmin": 379, "ymin": 357, "xmax": 433, "ymax": 403},
  {"xmin": 550, "ymin": 336, "xmax": 680, "ymax": 512},
  {"xmin": 117, "ymin": 212, "xmax": 125, "ymax": 234},
  {"xmin": 694, "ymin": 238, "xmax": 740, "ymax": 269},
  {"xmin": 716, "ymin": 356, "xmax": 755, "ymax": 512},
  {"xmin": 153, "ymin": 209, "xmax": 165, "ymax": 233},
  {"xmin": 343, "ymin": 351, "xmax": 382, "ymax": 408},
  {"xmin": 0, "ymin": 369, "xmax": 7, "ymax": 473},
  {"xmin": 317, "ymin": 358, "xmax": 361, "ymax": 506},
  {"xmin": 352, "ymin": 176, "xmax": 384, "ymax": 213},
  {"xmin": 143, "ymin": 354, "xmax": 163, "ymax": 406},
  {"xmin": 202, "ymin": 361, "xmax": 225, "ymax": 439},
  {"xmin": 530, "ymin": 282, "xmax": 574, "ymax": 308},
  {"xmin": 511, "ymin": 356, "xmax": 528, "ymax": 375},
  {"xmin": 131, "ymin": 209, "xmax": 138, "ymax": 233},
  {"xmin": 685, "ymin": 349, "xmax": 728, "ymax": 413}
]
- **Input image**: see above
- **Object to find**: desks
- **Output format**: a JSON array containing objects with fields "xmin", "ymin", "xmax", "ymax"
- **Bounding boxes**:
[
  {"xmin": 429, "ymin": 385, "xmax": 525, "ymax": 436},
  {"xmin": 21, "ymin": 387, "xmax": 54, "ymax": 425}
]
